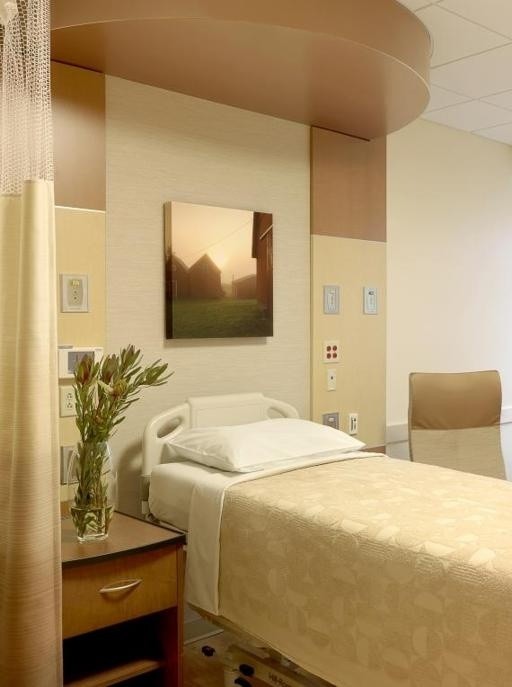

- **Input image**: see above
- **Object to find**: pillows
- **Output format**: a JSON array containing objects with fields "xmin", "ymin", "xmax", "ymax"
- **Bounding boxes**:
[{"xmin": 168, "ymin": 419, "xmax": 366, "ymax": 474}]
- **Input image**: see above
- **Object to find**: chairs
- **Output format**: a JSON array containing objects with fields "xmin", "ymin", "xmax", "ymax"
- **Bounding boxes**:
[{"xmin": 407, "ymin": 369, "xmax": 508, "ymax": 482}]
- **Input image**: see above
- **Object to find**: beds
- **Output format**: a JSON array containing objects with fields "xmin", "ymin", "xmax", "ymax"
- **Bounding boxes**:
[{"xmin": 140, "ymin": 391, "xmax": 510, "ymax": 686}]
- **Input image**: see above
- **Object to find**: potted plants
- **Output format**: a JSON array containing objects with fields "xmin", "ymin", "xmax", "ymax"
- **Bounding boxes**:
[{"xmin": 67, "ymin": 341, "xmax": 174, "ymax": 544}]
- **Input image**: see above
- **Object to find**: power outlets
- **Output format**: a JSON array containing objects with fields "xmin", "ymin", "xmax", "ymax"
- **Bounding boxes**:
[{"xmin": 60, "ymin": 385, "xmax": 97, "ymax": 417}]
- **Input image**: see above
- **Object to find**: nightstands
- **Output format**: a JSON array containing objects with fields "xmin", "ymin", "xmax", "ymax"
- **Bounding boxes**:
[{"xmin": 60, "ymin": 508, "xmax": 187, "ymax": 685}]
